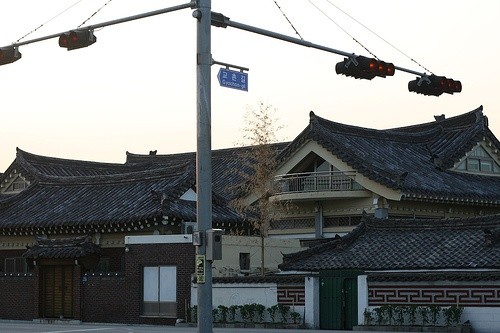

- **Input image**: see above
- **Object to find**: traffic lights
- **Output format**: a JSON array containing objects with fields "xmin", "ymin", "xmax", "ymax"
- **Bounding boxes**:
[
  {"xmin": 0, "ymin": 48, "xmax": 15, "ymax": 65},
  {"xmin": 439, "ymin": 77, "xmax": 462, "ymax": 92},
  {"xmin": 356, "ymin": 56, "xmax": 396, "ymax": 77},
  {"xmin": 58, "ymin": 30, "xmax": 89, "ymax": 49}
]
